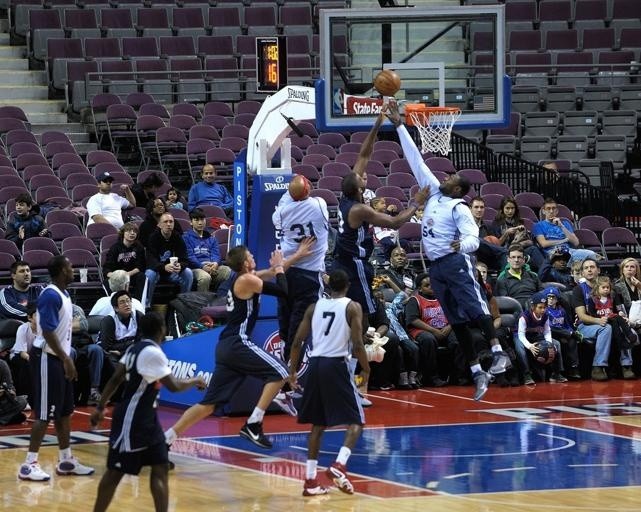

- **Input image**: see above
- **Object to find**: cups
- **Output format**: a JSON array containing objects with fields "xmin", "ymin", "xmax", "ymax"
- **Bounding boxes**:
[
  {"xmin": 164, "ymin": 335, "xmax": 174, "ymax": 342},
  {"xmin": 80, "ymin": 269, "xmax": 89, "ymax": 284}
]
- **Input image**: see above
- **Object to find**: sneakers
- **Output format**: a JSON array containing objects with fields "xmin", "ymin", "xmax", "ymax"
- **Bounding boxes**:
[
  {"xmin": 240, "ymin": 420, "xmax": 272, "ymax": 450},
  {"xmin": 488, "ymin": 351, "xmax": 519, "ymax": 386},
  {"xmin": 17, "ymin": 461, "xmax": 50, "ymax": 482},
  {"xmin": 56, "ymin": 457, "xmax": 93, "ymax": 476},
  {"xmin": 358, "ymin": 392, "xmax": 372, "ymax": 407},
  {"xmin": 523, "ymin": 364, "xmax": 581, "ymax": 384},
  {"xmin": 591, "ymin": 366, "xmax": 608, "ymax": 381},
  {"xmin": 273, "ymin": 389, "xmax": 297, "ymax": 417},
  {"xmin": 622, "ymin": 366, "xmax": 635, "ymax": 379},
  {"xmin": 78, "ymin": 388, "xmax": 111, "ymax": 407},
  {"xmin": 472, "ymin": 370, "xmax": 492, "ymax": 401},
  {"xmin": 326, "ymin": 460, "xmax": 356, "ymax": 495},
  {"xmin": 0, "ymin": 395, "xmax": 31, "ymax": 425},
  {"xmin": 302, "ymin": 478, "xmax": 329, "ymax": 496},
  {"xmin": 369, "ymin": 370, "xmax": 469, "ymax": 390}
]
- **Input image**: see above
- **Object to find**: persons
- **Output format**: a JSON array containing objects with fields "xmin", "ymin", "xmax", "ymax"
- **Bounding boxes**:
[
  {"xmin": 17, "ymin": 255, "xmax": 96, "ymax": 482},
  {"xmin": 144, "ymin": 235, "xmax": 320, "ymax": 470},
  {"xmin": 90, "ymin": 313, "xmax": 208, "ymax": 511},
  {"xmin": 289, "ymin": 268, "xmax": 370, "ymax": 497}
]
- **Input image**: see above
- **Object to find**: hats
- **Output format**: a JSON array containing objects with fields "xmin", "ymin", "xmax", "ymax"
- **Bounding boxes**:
[
  {"xmin": 543, "ymin": 287, "xmax": 560, "ymax": 298},
  {"xmin": 529, "ymin": 293, "xmax": 548, "ymax": 305},
  {"xmin": 98, "ymin": 172, "xmax": 114, "ymax": 182}
]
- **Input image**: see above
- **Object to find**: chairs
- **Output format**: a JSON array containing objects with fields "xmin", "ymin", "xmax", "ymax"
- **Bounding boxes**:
[{"xmin": 1, "ymin": 1, "xmax": 639, "ymax": 384}]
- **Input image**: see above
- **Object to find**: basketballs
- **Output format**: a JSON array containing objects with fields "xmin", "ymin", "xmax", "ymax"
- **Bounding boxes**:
[
  {"xmin": 375, "ymin": 70, "xmax": 401, "ymax": 95},
  {"xmin": 534, "ymin": 341, "xmax": 556, "ymax": 364}
]
